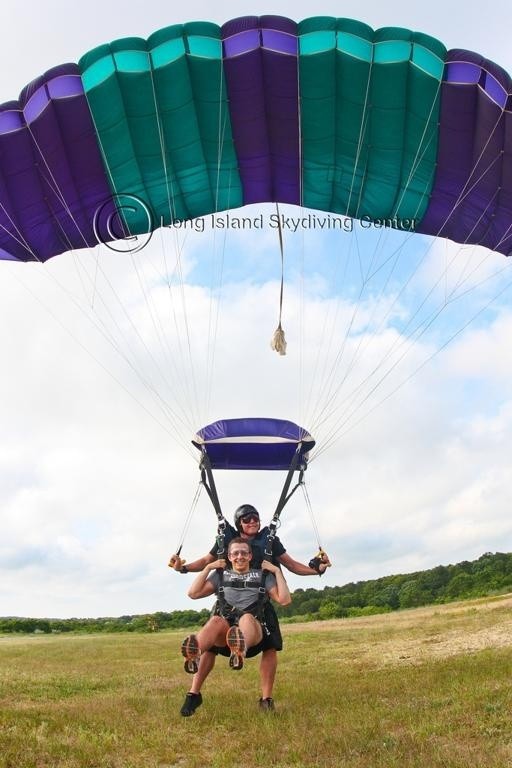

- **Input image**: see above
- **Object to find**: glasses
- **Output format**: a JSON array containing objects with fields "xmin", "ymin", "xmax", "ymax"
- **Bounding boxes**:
[
  {"xmin": 241, "ymin": 513, "xmax": 260, "ymax": 524},
  {"xmin": 229, "ymin": 551, "xmax": 250, "ymax": 558}
]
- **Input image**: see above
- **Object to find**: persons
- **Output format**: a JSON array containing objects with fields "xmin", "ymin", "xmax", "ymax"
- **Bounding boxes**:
[
  {"xmin": 168, "ymin": 503, "xmax": 332, "ymax": 717},
  {"xmin": 181, "ymin": 537, "xmax": 291, "ymax": 674}
]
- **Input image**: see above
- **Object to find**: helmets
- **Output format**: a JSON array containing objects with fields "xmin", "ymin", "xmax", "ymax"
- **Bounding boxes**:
[{"xmin": 234, "ymin": 504, "xmax": 259, "ymax": 531}]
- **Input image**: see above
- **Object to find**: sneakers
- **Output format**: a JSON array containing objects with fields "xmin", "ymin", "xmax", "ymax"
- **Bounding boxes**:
[
  {"xmin": 259, "ymin": 697, "xmax": 274, "ymax": 713},
  {"xmin": 225, "ymin": 626, "xmax": 245, "ymax": 671},
  {"xmin": 180, "ymin": 691, "xmax": 203, "ymax": 716},
  {"xmin": 181, "ymin": 634, "xmax": 199, "ymax": 674}
]
